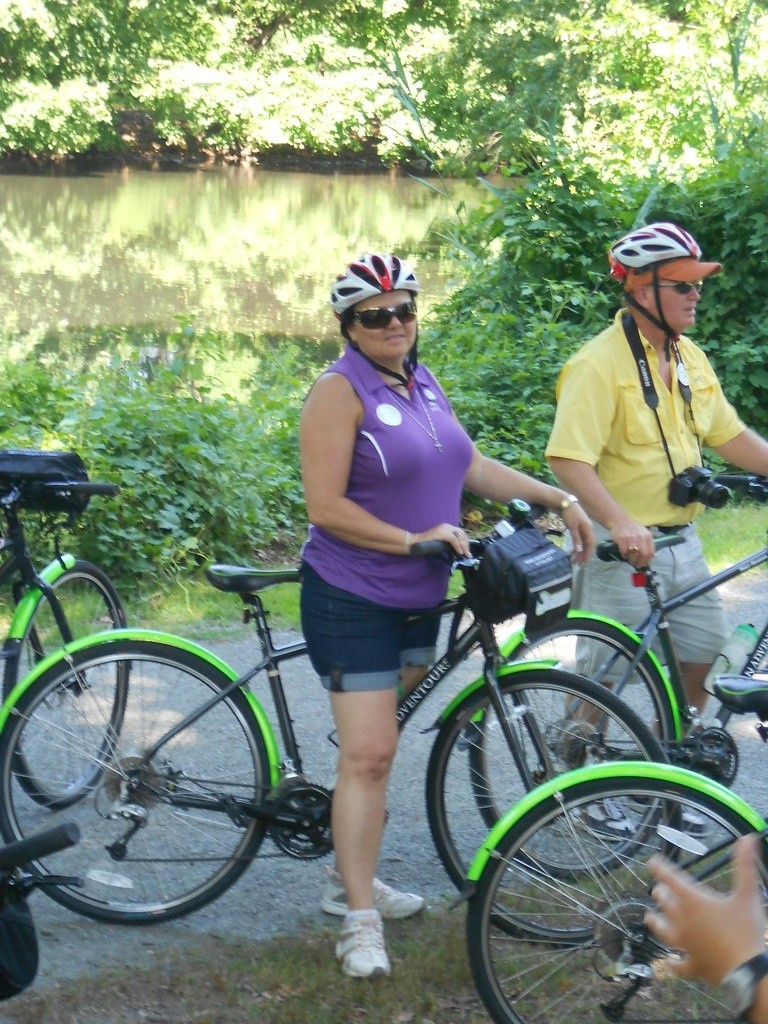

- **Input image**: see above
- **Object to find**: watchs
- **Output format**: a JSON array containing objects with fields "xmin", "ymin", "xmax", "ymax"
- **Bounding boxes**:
[{"xmin": 718, "ymin": 952, "xmax": 766, "ymax": 1022}]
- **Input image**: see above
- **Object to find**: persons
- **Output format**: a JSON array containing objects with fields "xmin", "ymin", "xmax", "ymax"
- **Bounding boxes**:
[{"xmin": 543, "ymin": 221, "xmax": 768, "ymax": 840}]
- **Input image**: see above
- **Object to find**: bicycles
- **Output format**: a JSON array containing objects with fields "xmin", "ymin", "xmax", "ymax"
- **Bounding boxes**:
[
  {"xmin": 1, "ymin": 476, "xmax": 133, "ymax": 810},
  {"xmin": 449, "ymin": 672, "xmax": 765, "ymax": 1023},
  {"xmin": 455, "ymin": 459, "xmax": 765, "ymax": 880},
  {"xmin": 2, "ymin": 500, "xmax": 685, "ymax": 950}
]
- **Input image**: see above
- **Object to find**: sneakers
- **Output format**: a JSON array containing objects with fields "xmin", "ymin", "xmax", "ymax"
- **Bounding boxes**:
[
  {"xmin": 320, "ymin": 862, "xmax": 425, "ymax": 921},
  {"xmin": 565, "ymin": 783, "xmax": 709, "ymax": 842},
  {"xmin": 334, "ymin": 913, "xmax": 391, "ymax": 982}
]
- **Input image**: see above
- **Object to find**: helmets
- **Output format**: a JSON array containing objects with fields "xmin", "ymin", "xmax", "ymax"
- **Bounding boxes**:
[
  {"xmin": 332, "ymin": 253, "xmax": 418, "ymax": 320},
  {"xmin": 610, "ymin": 219, "xmax": 703, "ymax": 283}
]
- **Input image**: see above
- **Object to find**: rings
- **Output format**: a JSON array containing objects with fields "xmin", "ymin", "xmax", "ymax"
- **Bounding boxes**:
[
  {"xmin": 454, "ymin": 530, "xmax": 460, "ymax": 537},
  {"xmin": 627, "ymin": 546, "xmax": 639, "ymax": 555}
]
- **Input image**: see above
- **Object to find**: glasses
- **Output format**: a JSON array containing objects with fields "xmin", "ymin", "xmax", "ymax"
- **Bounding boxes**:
[
  {"xmin": 344, "ymin": 301, "xmax": 418, "ymax": 329},
  {"xmin": 642, "ymin": 280, "xmax": 705, "ymax": 294}
]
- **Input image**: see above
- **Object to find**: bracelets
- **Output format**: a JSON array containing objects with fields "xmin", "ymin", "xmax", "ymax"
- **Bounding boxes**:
[
  {"xmin": 557, "ymin": 494, "xmax": 581, "ymax": 517},
  {"xmin": 403, "ymin": 529, "xmax": 414, "ymax": 556},
  {"xmin": 295, "ymin": 251, "xmax": 596, "ymax": 986}
]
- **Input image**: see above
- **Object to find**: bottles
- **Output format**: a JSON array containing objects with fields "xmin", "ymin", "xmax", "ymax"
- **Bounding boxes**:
[{"xmin": 701, "ymin": 623, "xmax": 759, "ymax": 696}]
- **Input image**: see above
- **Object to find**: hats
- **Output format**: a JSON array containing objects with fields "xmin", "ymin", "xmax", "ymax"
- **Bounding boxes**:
[{"xmin": 623, "ymin": 259, "xmax": 724, "ymax": 294}]
